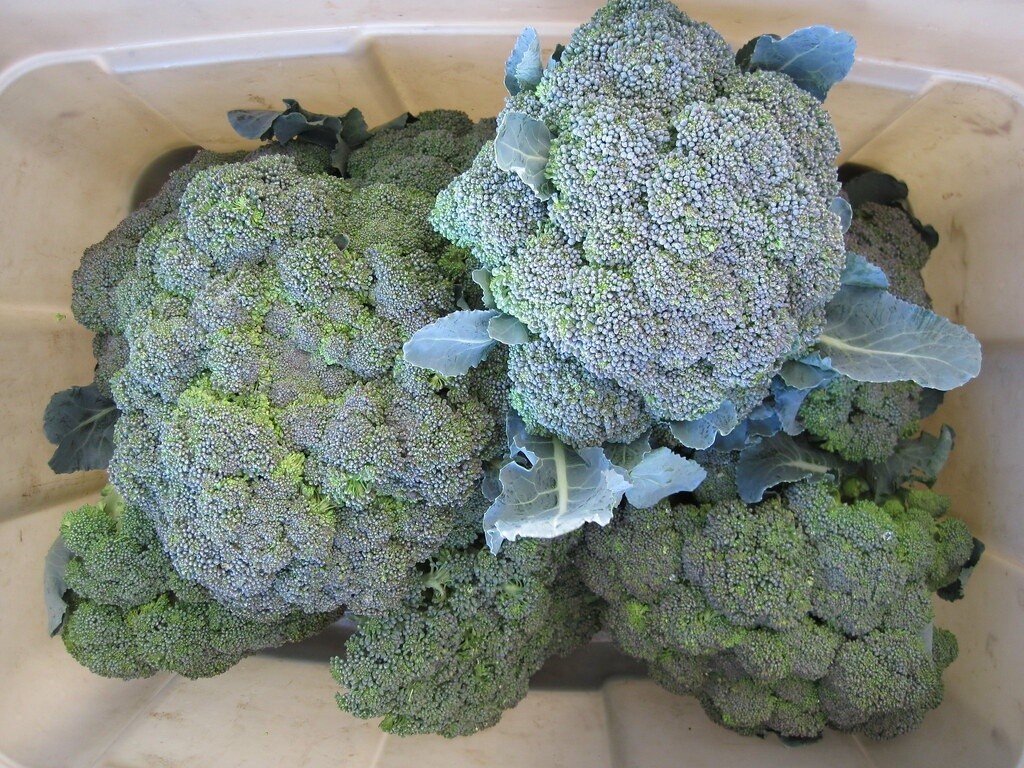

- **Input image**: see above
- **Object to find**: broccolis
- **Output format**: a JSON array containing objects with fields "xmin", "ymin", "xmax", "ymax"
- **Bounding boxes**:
[{"xmin": 46, "ymin": 0, "xmax": 984, "ymax": 739}]
